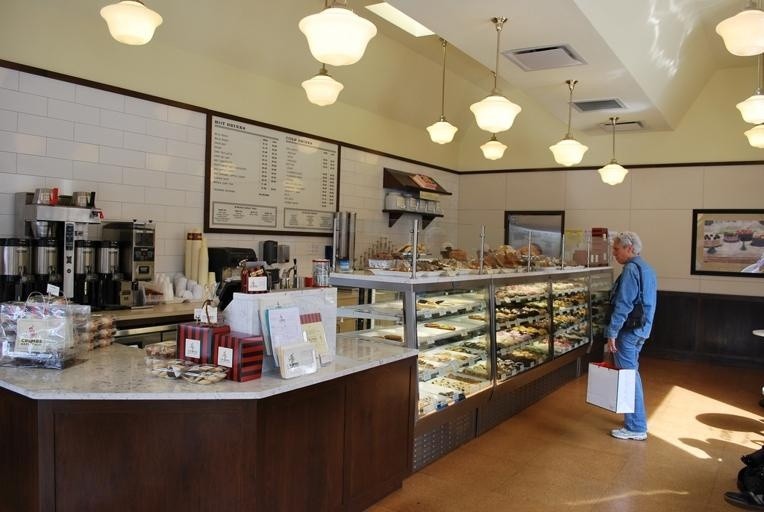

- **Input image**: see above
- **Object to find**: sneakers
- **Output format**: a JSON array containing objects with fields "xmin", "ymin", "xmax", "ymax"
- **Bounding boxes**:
[
  {"xmin": 611, "ymin": 427, "xmax": 647, "ymax": 440},
  {"xmin": 723, "ymin": 491, "xmax": 764, "ymax": 509}
]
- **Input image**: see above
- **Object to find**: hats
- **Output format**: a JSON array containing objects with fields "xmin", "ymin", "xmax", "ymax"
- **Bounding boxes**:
[{"xmin": 439, "ymin": 243, "xmax": 453, "ymax": 252}]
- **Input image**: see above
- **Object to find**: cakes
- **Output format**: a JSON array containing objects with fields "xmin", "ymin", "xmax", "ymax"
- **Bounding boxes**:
[
  {"xmin": 426, "ymin": 292, "xmax": 594, "ymax": 341},
  {"xmin": 418, "ymin": 319, "xmax": 593, "ymax": 417},
  {"xmin": 399, "ymin": 278, "xmax": 585, "ymax": 321},
  {"xmin": 415, "ymin": 306, "xmax": 588, "ymax": 384}
]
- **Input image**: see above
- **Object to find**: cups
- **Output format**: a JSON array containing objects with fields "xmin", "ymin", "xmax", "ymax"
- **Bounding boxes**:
[{"xmin": 155, "ymin": 226, "xmax": 216, "ymax": 300}]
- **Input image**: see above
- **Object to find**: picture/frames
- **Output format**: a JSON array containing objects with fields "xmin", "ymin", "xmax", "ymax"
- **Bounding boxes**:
[{"xmin": 690, "ymin": 209, "xmax": 764, "ymax": 278}]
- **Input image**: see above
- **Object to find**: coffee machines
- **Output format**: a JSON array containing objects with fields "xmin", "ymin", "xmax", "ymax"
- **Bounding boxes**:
[{"xmin": 0, "ymin": 237, "xmax": 121, "ymax": 309}]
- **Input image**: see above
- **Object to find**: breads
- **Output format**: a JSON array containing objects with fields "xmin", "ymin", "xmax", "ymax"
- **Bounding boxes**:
[{"xmin": 370, "ymin": 237, "xmax": 554, "ymax": 278}]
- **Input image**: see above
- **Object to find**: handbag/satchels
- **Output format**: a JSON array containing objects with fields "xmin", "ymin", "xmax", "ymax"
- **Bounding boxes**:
[
  {"xmin": 605, "ymin": 299, "xmax": 645, "ymax": 330},
  {"xmin": 585, "ymin": 361, "xmax": 637, "ymax": 414},
  {"xmin": 14, "ymin": 316, "xmax": 74, "ymax": 354}
]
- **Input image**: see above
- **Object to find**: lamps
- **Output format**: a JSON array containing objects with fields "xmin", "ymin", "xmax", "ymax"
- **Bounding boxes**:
[
  {"xmin": 735, "ymin": 56, "xmax": 764, "ymax": 125},
  {"xmin": 425, "ymin": 37, "xmax": 458, "ymax": 145},
  {"xmin": 597, "ymin": 117, "xmax": 629, "ymax": 186},
  {"xmin": 300, "ymin": 0, "xmax": 344, "ymax": 106},
  {"xmin": 99, "ymin": 0, "xmax": 162, "ymax": 46},
  {"xmin": 548, "ymin": 80, "xmax": 588, "ymax": 167},
  {"xmin": 479, "ymin": 72, "xmax": 509, "ymax": 161},
  {"xmin": 744, "ymin": 124, "xmax": 764, "ymax": 149},
  {"xmin": 298, "ymin": 0, "xmax": 377, "ymax": 66},
  {"xmin": 470, "ymin": 16, "xmax": 522, "ymax": 134},
  {"xmin": 714, "ymin": 0, "xmax": 764, "ymax": 57}
]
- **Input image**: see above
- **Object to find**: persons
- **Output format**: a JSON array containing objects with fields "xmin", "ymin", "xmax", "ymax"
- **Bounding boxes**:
[
  {"xmin": 602, "ymin": 231, "xmax": 658, "ymax": 441},
  {"xmin": 724, "ymin": 482, "xmax": 764, "ymax": 511},
  {"xmin": 440, "ymin": 244, "xmax": 455, "ymax": 259},
  {"xmin": 476, "ymin": 244, "xmax": 491, "ymax": 259}
]
